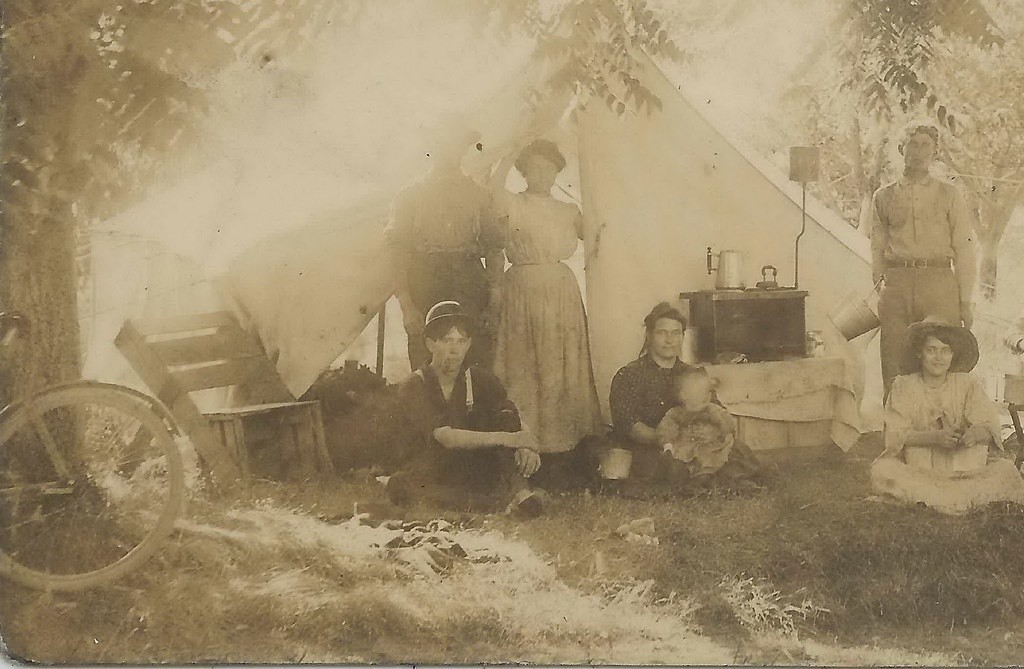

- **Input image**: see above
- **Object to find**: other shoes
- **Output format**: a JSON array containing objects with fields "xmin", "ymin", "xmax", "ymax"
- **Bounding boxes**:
[{"xmin": 504, "ymin": 488, "xmax": 548, "ymax": 520}]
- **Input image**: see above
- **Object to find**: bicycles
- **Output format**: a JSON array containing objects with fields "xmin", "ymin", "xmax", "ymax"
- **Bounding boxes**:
[{"xmin": 0, "ymin": 311, "xmax": 185, "ymax": 593}]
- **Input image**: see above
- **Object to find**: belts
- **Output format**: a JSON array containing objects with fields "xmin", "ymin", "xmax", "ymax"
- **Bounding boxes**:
[{"xmin": 884, "ymin": 258, "xmax": 953, "ymax": 269}]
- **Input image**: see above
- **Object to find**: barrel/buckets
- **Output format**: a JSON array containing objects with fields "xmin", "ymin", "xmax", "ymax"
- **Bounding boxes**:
[{"xmin": 829, "ymin": 290, "xmax": 880, "ymax": 342}]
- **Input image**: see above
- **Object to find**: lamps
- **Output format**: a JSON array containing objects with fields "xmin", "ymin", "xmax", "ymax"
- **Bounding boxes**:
[{"xmin": 788, "ymin": 145, "xmax": 820, "ymax": 290}]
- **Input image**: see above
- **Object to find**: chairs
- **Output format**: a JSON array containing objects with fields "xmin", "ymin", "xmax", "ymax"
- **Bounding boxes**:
[{"xmin": 113, "ymin": 306, "xmax": 335, "ymax": 498}]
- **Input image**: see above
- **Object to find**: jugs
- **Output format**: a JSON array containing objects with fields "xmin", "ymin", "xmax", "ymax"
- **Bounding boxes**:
[{"xmin": 707, "ymin": 247, "xmax": 748, "ymax": 289}]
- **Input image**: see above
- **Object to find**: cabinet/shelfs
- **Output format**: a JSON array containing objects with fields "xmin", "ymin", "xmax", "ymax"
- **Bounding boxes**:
[
  {"xmin": 715, "ymin": 356, "xmax": 845, "ymax": 450},
  {"xmin": 678, "ymin": 287, "xmax": 810, "ymax": 363}
]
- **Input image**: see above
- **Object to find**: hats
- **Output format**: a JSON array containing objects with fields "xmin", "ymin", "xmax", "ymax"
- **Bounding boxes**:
[
  {"xmin": 514, "ymin": 138, "xmax": 565, "ymax": 174},
  {"xmin": 901, "ymin": 313, "xmax": 981, "ymax": 372},
  {"xmin": 425, "ymin": 300, "xmax": 468, "ymax": 324}
]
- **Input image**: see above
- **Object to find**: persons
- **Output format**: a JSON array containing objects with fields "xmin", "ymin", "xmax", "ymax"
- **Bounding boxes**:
[
  {"xmin": 483, "ymin": 137, "xmax": 605, "ymax": 481},
  {"xmin": 390, "ymin": 108, "xmax": 499, "ymax": 378},
  {"xmin": 656, "ymin": 364, "xmax": 767, "ymax": 498},
  {"xmin": 871, "ymin": 314, "xmax": 1024, "ymax": 513},
  {"xmin": 606, "ymin": 300, "xmax": 726, "ymax": 498},
  {"xmin": 869, "ymin": 118, "xmax": 977, "ymax": 425},
  {"xmin": 392, "ymin": 298, "xmax": 548, "ymax": 521}
]
